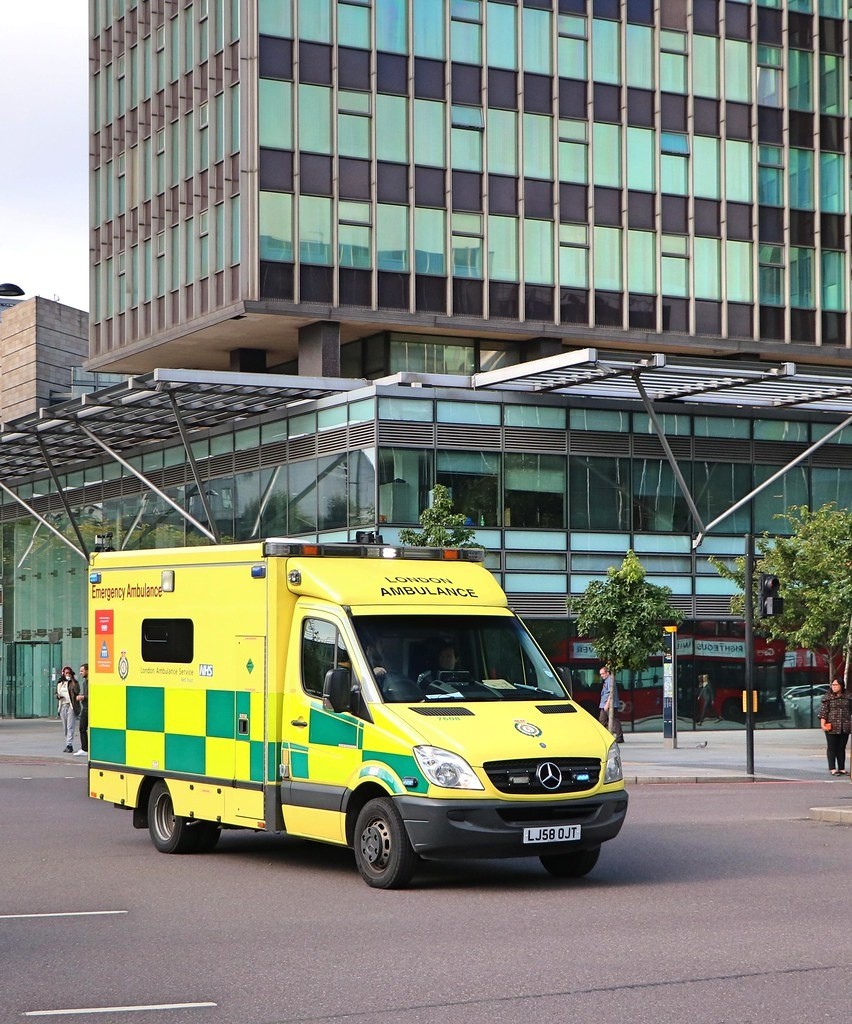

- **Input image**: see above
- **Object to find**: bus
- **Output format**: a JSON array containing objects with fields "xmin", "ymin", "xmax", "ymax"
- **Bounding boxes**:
[{"xmin": 561, "ymin": 635, "xmax": 843, "ymax": 729}]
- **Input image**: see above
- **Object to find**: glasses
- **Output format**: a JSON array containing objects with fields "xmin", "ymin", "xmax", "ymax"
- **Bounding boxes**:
[{"xmin": 830, "ymin": 683, "xmax": 840, "ymax": 688}]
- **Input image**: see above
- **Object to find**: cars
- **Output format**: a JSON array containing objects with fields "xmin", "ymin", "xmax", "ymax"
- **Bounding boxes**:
[{"xmin": 771, "ymin": 684, "xmax": 830, "ymax": 719}]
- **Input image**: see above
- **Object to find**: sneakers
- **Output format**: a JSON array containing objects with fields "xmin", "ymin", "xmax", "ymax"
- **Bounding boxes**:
[
  {"xmin": 73, "ymin": 749, "xmax": 88, "ymax": 755},
  {"xmin": 615, "ymin": 737, "xmax": 624, "ymax": 743}
]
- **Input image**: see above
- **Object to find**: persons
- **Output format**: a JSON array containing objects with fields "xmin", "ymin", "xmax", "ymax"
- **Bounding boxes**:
[
  {"xmin": 54, "ymin": 666, "xmax": 80, "ymax": 753},
  {"xmin": 597, "ymin": 667, "xmax": 625, "ymax": 744},
  {"xmin": 72, "ymin": 663, "xmax": 88, "ymax": 755},
  {"xmin": 347, "ymin": 638, "xmax": 387, "ymax": 688},
  {"xmin": 419, "ymin": 642, "xmax": 472, "ymax": 689},
  {"xmin": 817, "ymin": 677, "xmax": 852, "ymax": 776},
  {"xmin": 696, "ymin": 674, "xmax": 724, "ymax": 725}
]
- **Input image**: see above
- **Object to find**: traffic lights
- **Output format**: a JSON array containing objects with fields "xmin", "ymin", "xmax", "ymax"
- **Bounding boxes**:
[{"xmin": 760, "ymin": 575, "xmax": 785, "ymax": 618}]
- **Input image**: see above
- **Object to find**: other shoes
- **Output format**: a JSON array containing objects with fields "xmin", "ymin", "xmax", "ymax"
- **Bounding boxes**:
[
  {"xmin": 714, "ymin": 717, "xmax": 722, "ymax": 723},
  {"xmin": 839, "ymin": 770, "xmax": 848, "ymax": 774},
  {"xmin": 831, "ymin": 770, "xmax": 840, "ymax": 776},
  {"xmin": 697, "ymin": 722, "xmax": 702, "ymax": 725},
  {"xmin": 63, "ymin": 746, "xmax": 73, "ymax": 752}
]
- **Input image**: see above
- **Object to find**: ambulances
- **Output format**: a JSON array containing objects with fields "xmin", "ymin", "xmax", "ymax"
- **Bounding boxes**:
[{"xmin": 87, "ymin": 531, "xmax": 630, "ymax": 889}]
